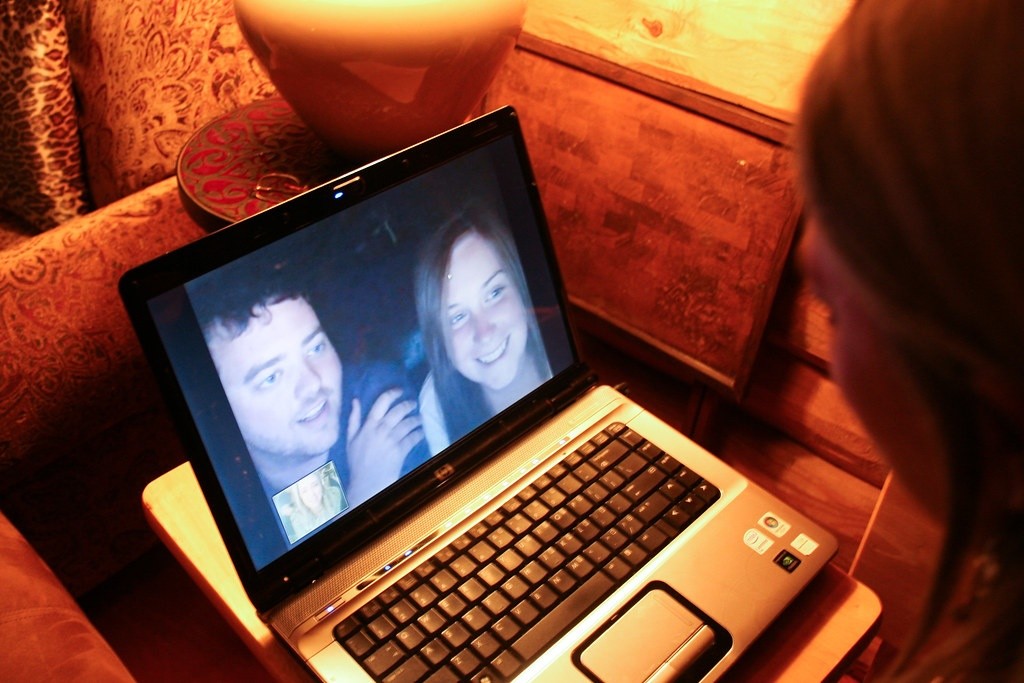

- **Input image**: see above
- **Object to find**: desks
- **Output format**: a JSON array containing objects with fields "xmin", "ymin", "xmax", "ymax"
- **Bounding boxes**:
[{"xmin": 140, "ymin": 461, "xmax": 883, "ymax": 683}]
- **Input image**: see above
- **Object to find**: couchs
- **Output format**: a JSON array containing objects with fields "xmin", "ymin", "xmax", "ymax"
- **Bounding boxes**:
[{"xmin": 0, "ymin": 0, "xmax": 282, "ymax": 598}]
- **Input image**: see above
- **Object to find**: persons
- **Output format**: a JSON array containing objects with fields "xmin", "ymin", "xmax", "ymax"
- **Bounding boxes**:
[
  {"xmin": 182, "ymin": 257, "xmax": 430, "ymax": 548},
  {"xmin": 792, "ymin": 0, "xmax": 1024, "ymax": 683},
  {"xmin": 417, "ymin": 206, "xmax": 554, "ymax": 460}
]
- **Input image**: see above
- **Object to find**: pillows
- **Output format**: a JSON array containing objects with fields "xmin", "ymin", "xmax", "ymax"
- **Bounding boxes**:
[{"xmin": 1, "ymin": 0, "xmax": 86, "ymax": 231}]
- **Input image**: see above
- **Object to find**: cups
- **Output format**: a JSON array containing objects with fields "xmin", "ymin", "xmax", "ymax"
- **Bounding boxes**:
[{"xmin": 232, "ymin": 1, "xmax": 534, "ymax": 156}]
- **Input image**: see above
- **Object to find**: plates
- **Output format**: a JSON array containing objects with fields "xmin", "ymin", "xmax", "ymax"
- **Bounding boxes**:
[{"xmin": 178, "ymin": 94, "xmax": 372, "ymax": 226}]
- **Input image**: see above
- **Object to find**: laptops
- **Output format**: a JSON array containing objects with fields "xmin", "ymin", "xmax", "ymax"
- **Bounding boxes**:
[{"xmin": 117, "ymin": 106, "xmax": 838, "ymax": 683}]
